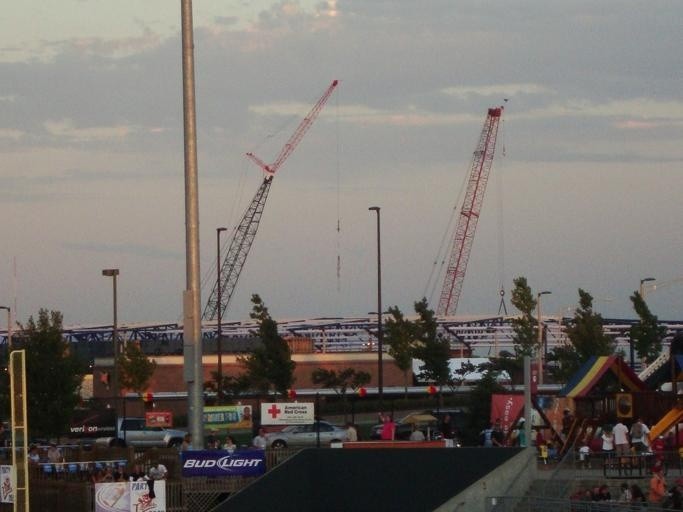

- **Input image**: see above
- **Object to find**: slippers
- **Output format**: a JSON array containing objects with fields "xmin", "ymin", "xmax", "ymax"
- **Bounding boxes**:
[{"xmin": 631, "ymin": 423, "xmax": 643, "ymax": 438}]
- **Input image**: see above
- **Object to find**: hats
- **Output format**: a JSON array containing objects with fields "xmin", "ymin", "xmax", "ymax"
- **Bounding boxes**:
[{"xmin": 251, "ymin": 420, "xmax": 352, "ymax": 452}]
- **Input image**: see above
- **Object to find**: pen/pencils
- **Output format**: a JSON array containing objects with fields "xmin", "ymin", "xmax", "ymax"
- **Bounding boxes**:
[{"xmin": 115, "ymin": 416, "xmax": 190, "ymax": 455}]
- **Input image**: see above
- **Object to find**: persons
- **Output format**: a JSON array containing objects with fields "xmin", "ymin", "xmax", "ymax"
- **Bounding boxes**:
[
  {"xmin": 532, "ymin": 408, "xmax": 683, "ymax": 512},
  {"xmin": 0, "ymin": 422, "xmax": 10, "ymax": 446},
  {"xmin": 252, "ymin": 428, "xmax": 268, "ymax": 450},
  {"xmin": 240, "ymin": 407, "xmax": 251, "ymax": 422},
  {"xmin": 182, "ymin": 434, "xmax": 192, "ymax": 451},
  {"xmin": 439, "ymin": 414, "xmax": 452, "ymax": 434},
  {"xmin": 28, "ymin": 439, "xmax": 169, "ymax": 483},
  {"xmin": 409, "ymin": 425, "xmax": 426, "ymax": 441},
  {"xmin": 378, "ymin": 412, "xmax": 397, "ymax": 441},
  {"xmin": 346, "ymin": 423, "xmax": 358, "ymax": 441},
  {"xmin": 208, "ymin": 430, "xmax": 237, "ymax": 454},
  {"xmin": 490, "ymin": 417, "xmax": 526, "ymax": 447}
]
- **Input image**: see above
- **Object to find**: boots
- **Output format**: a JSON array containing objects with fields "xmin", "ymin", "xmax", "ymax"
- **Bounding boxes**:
[{"xmin": 369, "ymin": 409, "xmax": 476, "ymax": 443}]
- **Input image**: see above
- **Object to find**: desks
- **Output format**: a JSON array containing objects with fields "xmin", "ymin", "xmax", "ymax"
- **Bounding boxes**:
[
  {"xmin": 368, "ymin": 207, "xmax": 383, "ymax": 400},
  {"xmin": 216, "ymin": 227, "xmax": 226, "ymax": 399},
  {"xmin": 537, "ymin": 290, "xmax": 550, "ymax": 385},
  {"xmin": 638, "ymin": 277, "xmax": 655, "ymax": 324},
  {"xmin": 101, "ymin": 269, "xmax": 119, "ymax": 416},
  {"xmin": 0, "ymin": 307, "xmax": 12, "ymax": 387}
]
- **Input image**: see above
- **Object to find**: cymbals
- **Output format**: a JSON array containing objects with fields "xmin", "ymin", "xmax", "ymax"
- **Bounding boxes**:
[
  {"xmin": 196, "ymin": 82, "xmax": 340, "ymax": 324},
  {"xmin": 419, "ymin": 98, "xmax": 511, "ymax": 318}
]
